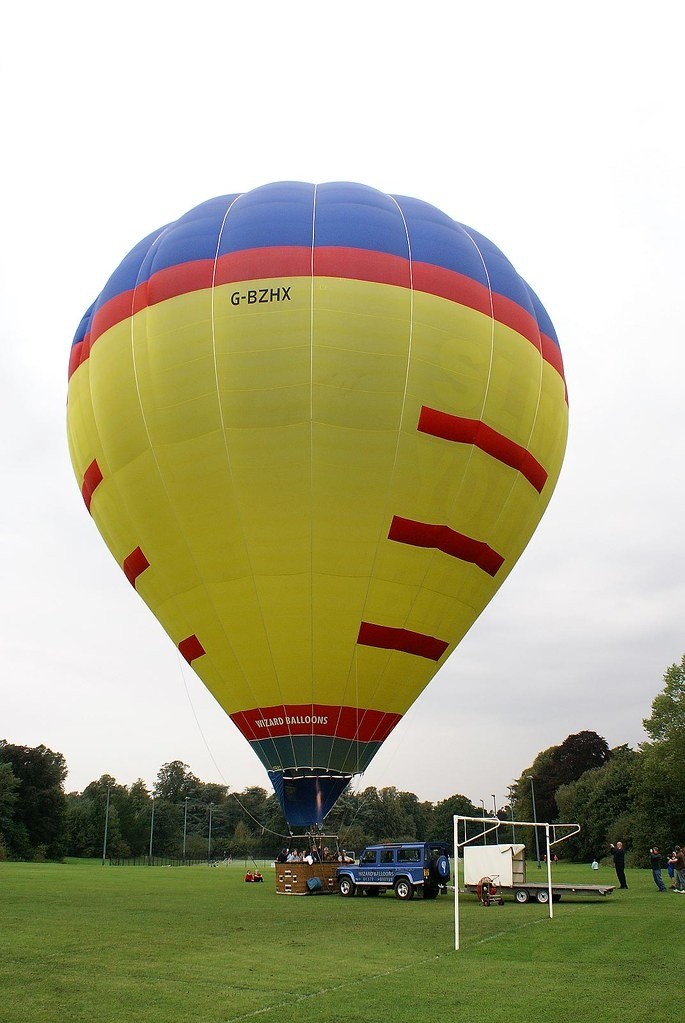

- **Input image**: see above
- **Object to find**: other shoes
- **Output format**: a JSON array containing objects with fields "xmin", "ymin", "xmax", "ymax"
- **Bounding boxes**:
[
  {"xmin": 669, "ymin": 885, "xmax": 675, "ymax": 890},
  {"xmin": 673, "ymin": 889, "xmax": 685, "ymax": 894},
  {"xmin": 617, "ymin": 885, "xmax": 628, "ymax": 889},
  {"xmin": 656, "ymin": 889, "xmax": 667, "ymax": 892}
]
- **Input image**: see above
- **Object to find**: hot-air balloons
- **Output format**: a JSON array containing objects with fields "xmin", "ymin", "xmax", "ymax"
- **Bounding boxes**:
[{"xmin": 63, "ymin": 178, "xmax": 571, "ymax": 900}]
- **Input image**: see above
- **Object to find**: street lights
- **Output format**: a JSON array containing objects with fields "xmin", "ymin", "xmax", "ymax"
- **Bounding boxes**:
[
  {"xmin": 101, "ymin": 784, "xmax": 110, "ymax": 865},
  {"xmin": 148, "ymin": 791, "xmax": 157, "ymax": 867},
  {"xmin": 526, "ymin": 775, "xmax": 542, "ymax": 869},
  {"xmin": 480, "ymin": 799, "xmax": 486, "ymax": 845},
  {"xmin": 507, "ymin": 786, "xmax": 516, "ymax": 844},
  {"xmin": 181, "ymin": 796, "xmax": 190, "ymax": 866},
  {"xmin": 491, "ymin": 794, "xmax": 499, "ymax": 845},
  {"xmin": 207, "ymin": 802, "xmax": 215, "ymax": 863}
]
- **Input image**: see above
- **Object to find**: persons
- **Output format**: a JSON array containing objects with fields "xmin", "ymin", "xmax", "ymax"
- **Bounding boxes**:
[
  {"xmin": 610, "ymin": 842, "xmax": 628, "ymax": 889},
  {"xmin": 666, "ymin": 845, "xmax": 685, "ymax": 894},
  {"xmin": 544, "ymin": 855, "xmax": 547, "ymax": 862},
  {"xmin": 253, "ymin": 869, "xmax": 264, "ymax": 882},
  {"xmin": 592, "ymin": 859, "xmax": 598, "ymax": 870},
  {"xmin": 277, "ymin": 845, "xmax": 354, "ymax": 865},
  {"xmin": 554, "ymin": 855, "xmax": 558, "ymax": 864},
  {"xmin": 244, "ymin": 869, "xmax": 254, "ymax": 882},
  {"xmin": 650, "ymin": 847, "xmax": 666, "ymax": 892}
]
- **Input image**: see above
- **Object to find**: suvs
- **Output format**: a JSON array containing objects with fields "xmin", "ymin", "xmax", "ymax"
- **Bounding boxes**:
[{"xmin": 334, "ymin": 840, "xmax": 452, "ymax": 900}]
